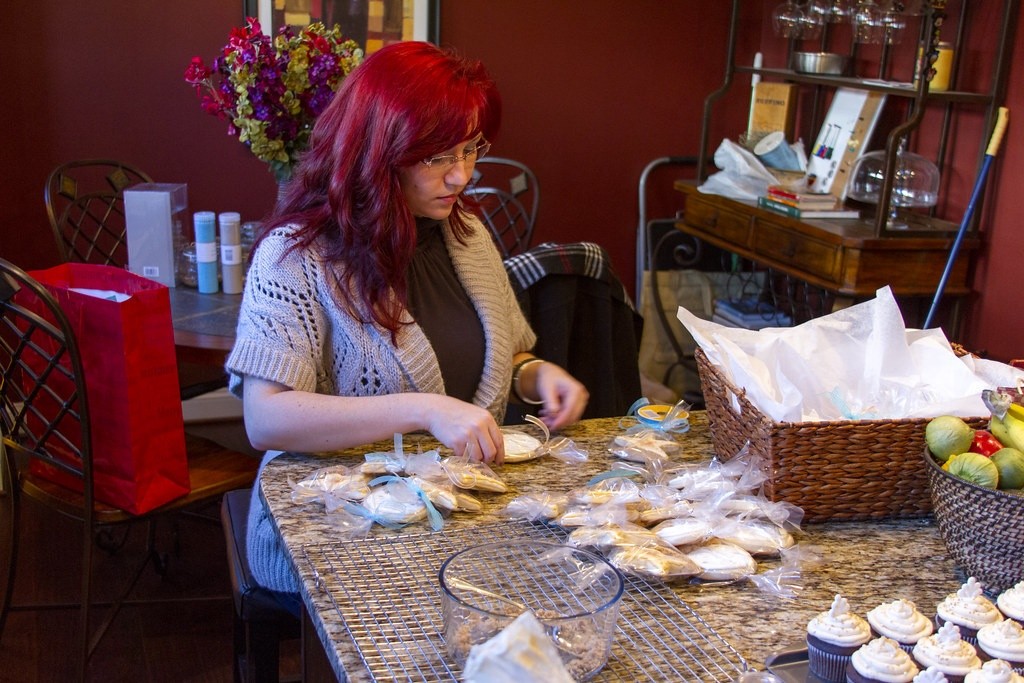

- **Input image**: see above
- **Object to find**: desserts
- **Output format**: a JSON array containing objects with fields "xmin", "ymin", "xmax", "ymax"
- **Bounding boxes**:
[{"xmin": 806, "ymin": 575, "xmax": 1024, "ymax": 683}]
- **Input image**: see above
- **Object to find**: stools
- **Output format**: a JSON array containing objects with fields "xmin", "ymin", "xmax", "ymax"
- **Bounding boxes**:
[{"xmin": 225, "ymin": 488, "xmax": 313, "ymax": 683}]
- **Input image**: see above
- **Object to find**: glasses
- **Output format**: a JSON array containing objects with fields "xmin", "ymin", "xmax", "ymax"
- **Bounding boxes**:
[{"xmin": 419, "ymin": 134, "xmax": 492, "ymax": 175}]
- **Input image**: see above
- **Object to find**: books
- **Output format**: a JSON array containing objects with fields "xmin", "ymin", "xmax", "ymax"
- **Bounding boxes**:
[
  {"xmin": 712, "ymin": 297, "xmax": 791, "ymax": 330},
  {"xmin": 757, "ymin": 185, "xmax": 860, "ymax": 219}
]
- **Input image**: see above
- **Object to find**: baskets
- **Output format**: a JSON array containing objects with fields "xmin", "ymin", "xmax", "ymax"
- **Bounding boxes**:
[
  {"xmin": 693, "ymin": 342, "xmax": 997, "ymax": 526},
  {"xmin": 924, "ymin": 431, "xmax": 1024, "ymax": 600}
]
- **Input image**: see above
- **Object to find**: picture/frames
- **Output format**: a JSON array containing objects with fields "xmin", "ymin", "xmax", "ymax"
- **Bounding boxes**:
[{"xmin": 244, "ymin": 0, "xmax": 442, "ymax": 63}]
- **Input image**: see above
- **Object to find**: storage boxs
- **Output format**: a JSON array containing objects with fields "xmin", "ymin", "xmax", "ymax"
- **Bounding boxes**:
[{"xmin": 122, "ymin": 180, "xmax": 190, "ymax": 289}]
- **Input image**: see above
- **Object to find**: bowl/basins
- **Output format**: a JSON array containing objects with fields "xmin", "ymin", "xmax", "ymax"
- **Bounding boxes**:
[{"xmin": 437, "ymin": 540, "xmax": 624, "ymax": 681}]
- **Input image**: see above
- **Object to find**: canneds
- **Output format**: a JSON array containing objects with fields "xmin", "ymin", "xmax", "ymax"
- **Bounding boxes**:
[{"xmin": 913, "ymin": 41, "xmax": 953, "ymax": 93}]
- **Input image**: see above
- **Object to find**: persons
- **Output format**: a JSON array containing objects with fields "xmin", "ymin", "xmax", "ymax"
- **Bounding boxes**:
[{"xmin": 223, "ymin": 42, "xmax": 590, "ymax": 620}]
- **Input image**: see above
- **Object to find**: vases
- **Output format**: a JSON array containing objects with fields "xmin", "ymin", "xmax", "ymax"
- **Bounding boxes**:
[{"xmin": 276, "ymin": 178, "xmax": 293, "ymax": 200}]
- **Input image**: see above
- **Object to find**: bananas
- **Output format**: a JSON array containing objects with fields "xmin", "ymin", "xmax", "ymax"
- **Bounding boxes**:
[{"xmin": 981, "ymin": 390, "xmax": 1024, "ymax": 455}]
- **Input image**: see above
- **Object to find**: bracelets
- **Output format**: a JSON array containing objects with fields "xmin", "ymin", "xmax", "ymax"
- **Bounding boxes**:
[{"xmin": 511, "ymin": 358, "xmax": 545, "ymax": 406}]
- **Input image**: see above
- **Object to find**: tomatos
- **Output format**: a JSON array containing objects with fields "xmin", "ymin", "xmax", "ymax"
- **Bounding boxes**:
[{"xmin": 969, "ymin": 430, "xmax": 1003, "ymax": 457}]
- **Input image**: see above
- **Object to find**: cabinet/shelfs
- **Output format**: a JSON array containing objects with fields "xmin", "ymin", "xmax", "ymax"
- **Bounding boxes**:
[{"xmin": 665, "ymin": 0, "xmax": 1021, "ymax": 344}]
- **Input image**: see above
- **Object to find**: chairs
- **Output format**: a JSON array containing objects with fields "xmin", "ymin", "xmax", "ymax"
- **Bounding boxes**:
[
  {"xmin": 464, "ymin": 158, "xmax": 540, "ymax": 259},
  {"xmin": 0, "ymin": 257, "xmax": 264, "ymax": 683},
  {"xmin": 503, "ymin": 242, "xmax": 646, "ymax": 410},
  {"xmin": 43, "ymin": 159, "xmax": 157, "ymax": 271}
]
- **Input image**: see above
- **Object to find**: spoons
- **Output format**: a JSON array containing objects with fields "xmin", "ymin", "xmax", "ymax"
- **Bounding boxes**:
[{"xmin": 447, "ymin": 573, "xmax": 585, "ymax": 657}]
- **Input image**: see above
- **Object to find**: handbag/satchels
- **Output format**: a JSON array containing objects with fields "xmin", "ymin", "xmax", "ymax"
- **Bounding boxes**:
[{"xmin": 10, "ymin": 261, "xmax": 196, "ymax": 518}]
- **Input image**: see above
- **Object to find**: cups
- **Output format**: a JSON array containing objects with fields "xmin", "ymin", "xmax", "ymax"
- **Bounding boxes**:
[
  {"xmin": 754, "ymin": 132, "xmax": 800, "ymax": 171},
  {"xmin": 771, "ymin": 0, "xmax": 933, "ymax": 43}
]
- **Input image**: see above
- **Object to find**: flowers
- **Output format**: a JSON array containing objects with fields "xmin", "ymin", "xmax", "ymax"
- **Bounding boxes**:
[{"xmin": 186, "ymin": 19, "xmax": 364, "ymax": 182}]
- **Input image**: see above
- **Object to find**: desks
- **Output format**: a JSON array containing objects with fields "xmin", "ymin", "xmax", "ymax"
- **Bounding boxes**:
[
  {"xmin": 118, "ymin": 274, "xmax": 245, "ymax": 368},
  {"xmin": 257, "ymin": 413, "xmax": 1024, "ymax": 683}
]
